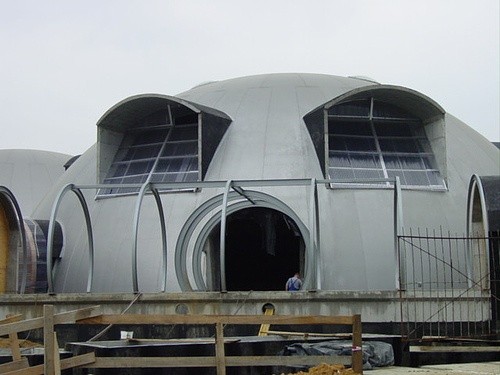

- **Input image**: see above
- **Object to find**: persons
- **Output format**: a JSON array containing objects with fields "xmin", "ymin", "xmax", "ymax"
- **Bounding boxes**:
[{"xmin": 285, "ymin": 272, "xmax": 303, "ymax": 291}]
